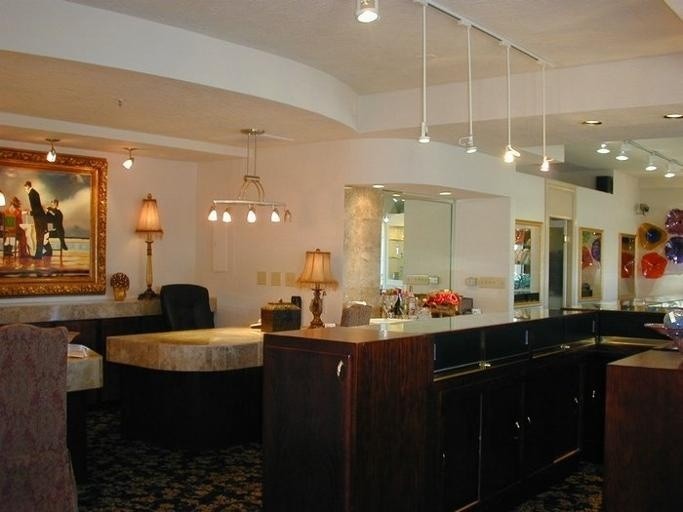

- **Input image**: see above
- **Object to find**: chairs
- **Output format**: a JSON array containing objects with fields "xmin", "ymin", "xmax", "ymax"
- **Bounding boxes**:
[
  {"xmin": 159, "ymin": 284, "xmax": 215, "ymax": 331},
  {"xmin": 0, "ymin": 322, "xmax": 81, "ymax": 512}
]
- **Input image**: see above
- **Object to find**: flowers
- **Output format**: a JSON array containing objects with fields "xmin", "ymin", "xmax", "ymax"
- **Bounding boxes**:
[
  {"xmin": 109, "ymin": 271, "xmax": 130, "ymax": 290},
  {"xmin": 424, "ymin": 292, "xmax": 461, "ymax": 307},
  {"xmin": 425, "ymin": 292, "xmax": 459, "ymax": 308}
]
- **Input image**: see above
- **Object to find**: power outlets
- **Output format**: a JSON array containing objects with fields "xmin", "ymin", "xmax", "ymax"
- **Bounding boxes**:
[
  {"xmin": 478, "ymin": 275, "xmax": 505, "ymax": 291},
  {"xmin": 402, "ymin": 274, "xmax": 430, "ymax": 288}
]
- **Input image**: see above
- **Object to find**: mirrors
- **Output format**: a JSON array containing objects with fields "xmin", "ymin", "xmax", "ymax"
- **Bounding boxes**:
[
  {"xmin": 514, "ymin": 219, "xmax": 544, "ymax": 309},
  {"xmin": 577, "ymin": 225, "xmax": 605, "ymax": 305},
  {"xmin": 616, "ymin": 231, "xmax": 639, "ymax": 303}
]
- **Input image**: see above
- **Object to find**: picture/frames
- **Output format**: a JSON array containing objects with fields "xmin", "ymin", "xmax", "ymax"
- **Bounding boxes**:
[{"xmin": 0, "ymin": 144, "xmax": 111, "ymax": 299}]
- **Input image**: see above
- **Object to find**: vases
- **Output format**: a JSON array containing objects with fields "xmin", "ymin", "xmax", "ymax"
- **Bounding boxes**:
[
  {"xmin": 428, "ymin": 303, "xmax": 455, "ymax": 319},
  {"xmin": 114, "ymin": 287, "xmax": 126, "ymax": 301}
]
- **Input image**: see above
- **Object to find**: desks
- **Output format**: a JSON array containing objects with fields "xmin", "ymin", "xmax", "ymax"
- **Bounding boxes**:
[
  {"xmin": 105, "ymin": 326, "xmax": 266, "ymax": 453},
  {"xmin": 67, "ymin": 343, "xmax": 104, "ymax": 393}
]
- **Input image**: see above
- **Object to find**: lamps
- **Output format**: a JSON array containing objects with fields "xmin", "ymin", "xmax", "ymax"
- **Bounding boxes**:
[
  {"xmin": 415, "ymin": 0, "xmax": 555, "ymax": 175},
  {"xmin": 292, "ymin": 246, "xmax": 339, "ymax": 330},
  {"xmin": 596, "ymin": 143, "xmax": 611, "ymax": 155},
  {"xmin": 581, "ymin": 120, "xmax": 603, "ymax": 126},
  {"xmin": 664, "ymin": 113, "xmax": 683, "ymax": 120},
  {"xmin": 207, "ymin": 128, "xmax": 293, "ymax": 226},
  {"xmin": 122, "ymin": 146, "xmax": 139, "ymax": 172},
  {"xmin": 354, "ymin": 2, "xmax": 381, "ymax": 24},
  {"xmin": 132, "ymin": 192, "xmax": 165, "ymax": 301},
  {"xmin": 615, "ymin": 138, "xmax": 683, "ymax": 180},
  {"xmin": 45, "ymin": 137, "xmax": 62, "ymax": 164}
]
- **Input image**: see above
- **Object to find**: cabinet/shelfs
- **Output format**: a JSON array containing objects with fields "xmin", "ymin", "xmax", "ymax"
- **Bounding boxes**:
[
  {"xmin": 262, "ymin": 301, "xmax": 683, "ymax": 512},
  {"xmin": 1, "ymin": 296, "xmax": 218, "ymax": 326}
]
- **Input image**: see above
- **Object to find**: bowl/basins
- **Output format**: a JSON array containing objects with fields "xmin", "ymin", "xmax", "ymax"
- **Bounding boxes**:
[{"xmin": 644, "ymin": 323, "xmax": 683, "ymax": 354}]
